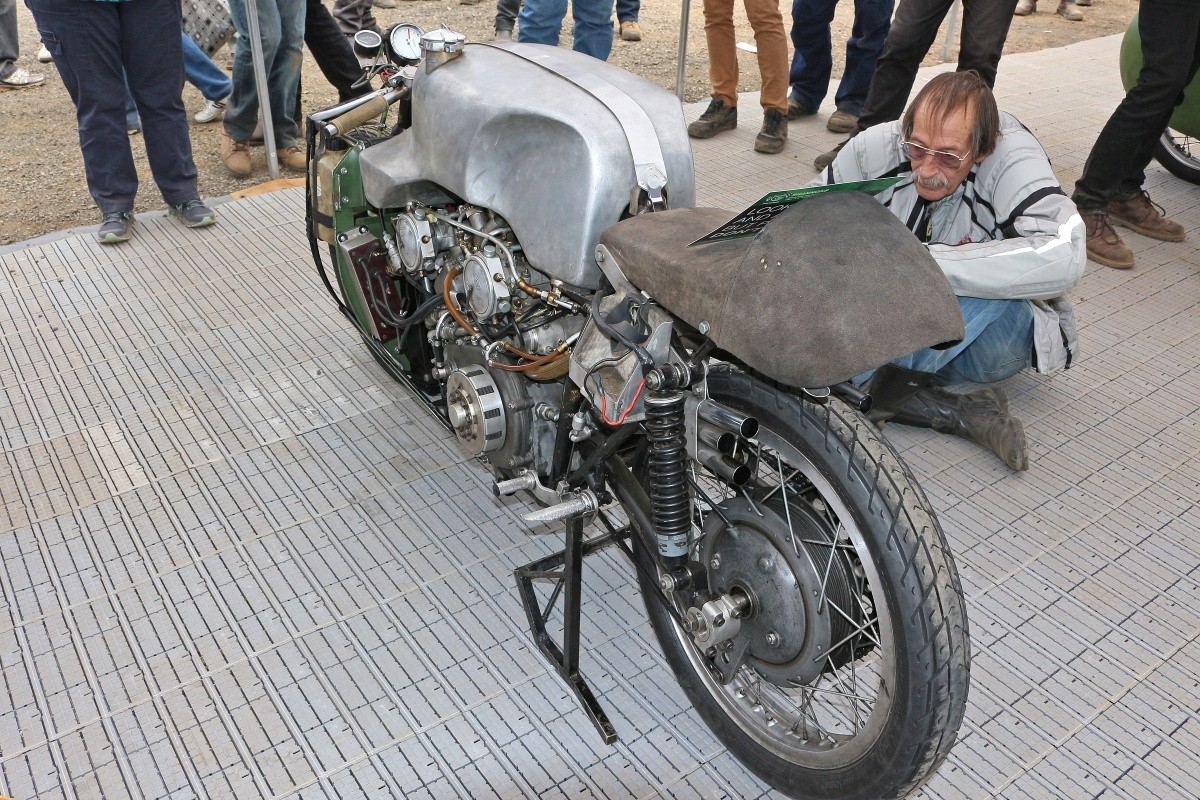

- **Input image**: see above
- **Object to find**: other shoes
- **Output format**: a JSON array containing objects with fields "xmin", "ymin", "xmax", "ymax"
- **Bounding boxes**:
[
  {"xmin": 827, "ymin": 110, "xmax": 859, "ymax": 131},
  {"xmin": 249, "ymin": 111, "xmax": 303, "ymax": 146},
  {"xmin": 1057, "ymin": 2, "xmax": 1083, "ymax": 21},
  {"xmin": 814, "ymin": 137, "xmax": 855, "ymax": 171},
  {"xmin": 496, "ymin": 27, "xmax": 514, "ymax": 43},
  {"xmin": 1014, "ymin": 0, "xmax": 1036, "ymax": 15},
  {"xmin": 784, "ymin": 97, "xmax": 820, "ymax": 120}
]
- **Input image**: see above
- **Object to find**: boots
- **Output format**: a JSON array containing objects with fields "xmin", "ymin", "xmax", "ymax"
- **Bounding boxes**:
[{"xmin": 863, "ymin": 361, "xmax": 1030, "ymax": 473}]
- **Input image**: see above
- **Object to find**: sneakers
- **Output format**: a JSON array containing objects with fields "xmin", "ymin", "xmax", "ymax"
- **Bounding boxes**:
[
  {"xmin": 38, "ymin": 42, "xmax": 54, "ymax": 62},
  {"xmin": 168, "ymin": 197, "xmax": 215, "ymax": 228},
  {"xmin": 1080, "ymin": 207, "xmax": 1136, "ymax": 269},
  {"xmin": 754, "ymin": 107, "xmax": 789, "ymax": 153},
  {"xmin": 687, "ymin": 95, "xmax": 738, "ymax": 138},
  {"xmin": 0, "ymin": 64, "xmax": 45, "ymax": 89},
  {"xmin": 96, "ymin": 208, "xmax": 136, "ymax": 244},
  {"xmin": 1109, "ymin": 187, "xmax": 1184, "ymax": 242},
  {"xmin": 221, "ymin": 128, "xmax": 253, "ymax": 175},
  {"xmin": 620, "ymin": 20, "xmax": 640, "ymax": 41},
  {"xmin": 275, "ymin": 145, "xmax": 310, "ymax": 173},
  {"xmin": 194, "ymin": 88, "xmax": 232, "ymax": 123}
]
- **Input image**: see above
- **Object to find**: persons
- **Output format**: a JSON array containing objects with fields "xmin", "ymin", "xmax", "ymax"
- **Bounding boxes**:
[
  {"xmin": 1071, "ymin": 0, "xmax": 1200, "ymax": 268},
  {"xmin": 332, "ymin": 0, "xmax": 388, "ymax": 48},
  {"xmin": 493, "ymin": 0, "xmax": 522, "ymax": 42},
  {"xmin": 26, "ymin": 0, "xmax": 219, "ymax": 244},
  {"xmin": 795, "ymin": 69, "xmax": 1089, "ymax": 472},
  {"xmin": 286, "ymin": 0, "xmax": 378, "ymax": 132},
  {"xmin": 813, "ymin": 0, "xmax": 1017, "ymax": 173},
  {"xmin": 687, "ymin": 0, "xmax": 790, "ymax": 154},
  {"xmin": 1014, "ymin": 0, "xmax": 1095, "ymax": 21},
  {"xmin": 223, "ymin": 0, "xmax": 310, "ymax": 175},
  {"xmin": 0, "ymin": 0, "xmax": 53, "ymax": 90},
  {"xmin": 616, "ymin": 0, "xmax": 640, "ymax": 41},
  {"xmin": 518, "ymin": 0, "xmax": 614, "ymax": 62},
  {"xmin": 124, "ymin": 0, "xmax": 236, "ymax": 135},
  {"xmin": 786, "ymin": 0, "xmax": 894, "ymax": 133}
]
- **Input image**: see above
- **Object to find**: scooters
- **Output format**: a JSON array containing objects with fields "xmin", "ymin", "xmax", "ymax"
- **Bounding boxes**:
[{"xmin": 1119, "ymin": 9, "xmax": 1200, "ymax": 186}]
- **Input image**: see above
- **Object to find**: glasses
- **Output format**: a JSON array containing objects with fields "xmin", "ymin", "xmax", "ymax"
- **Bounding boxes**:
[{"xmin": 901, "ymin": 127, "xmax": 973, "ymax": 171}]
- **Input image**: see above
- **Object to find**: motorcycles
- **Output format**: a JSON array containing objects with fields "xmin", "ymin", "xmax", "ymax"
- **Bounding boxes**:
[{"xmin": 302, "ymin": 20, "xmax": 974, "ymax": 799}]
